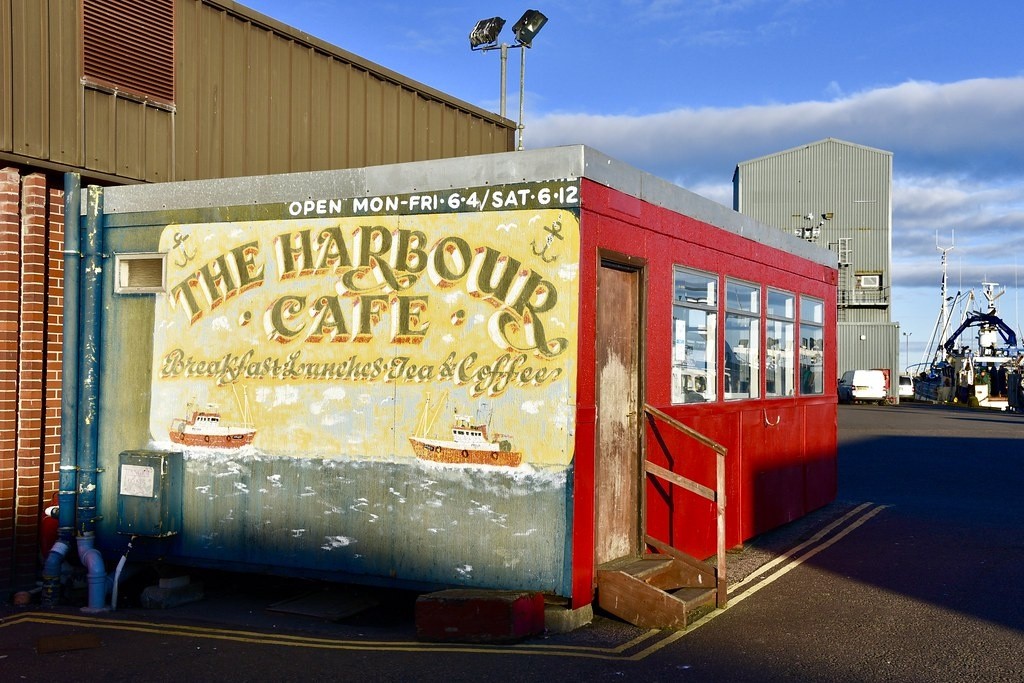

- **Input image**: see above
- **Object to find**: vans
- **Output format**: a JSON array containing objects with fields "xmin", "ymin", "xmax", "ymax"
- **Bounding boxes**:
[
  {"xmin": 897, "ymin": 374, "xmax": 916, "ymax": 402},
  {"xmin": 837, "ymin": 369, "xmax": 888, "ymax": 406}
]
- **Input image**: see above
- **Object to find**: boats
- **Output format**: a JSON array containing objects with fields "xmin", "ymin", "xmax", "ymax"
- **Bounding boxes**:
[{"xmin": 905, "ymin": 223, "xmax": 1024, "ymax": 415}]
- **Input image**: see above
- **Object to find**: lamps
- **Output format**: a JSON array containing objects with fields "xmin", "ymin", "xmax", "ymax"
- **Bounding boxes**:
[{"xmin": 470, "ymin": 9, "xmax": 549, "ymax": 118}]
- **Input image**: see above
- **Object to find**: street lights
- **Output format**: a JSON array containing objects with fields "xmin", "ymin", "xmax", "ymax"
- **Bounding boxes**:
[
  {"xmin": 903, "ymin": 332, "xmax": 912, "ymax": 376},
  {"xmin": 468, "ymin": 9, "xmax": 549, "ymax": 120}
]
